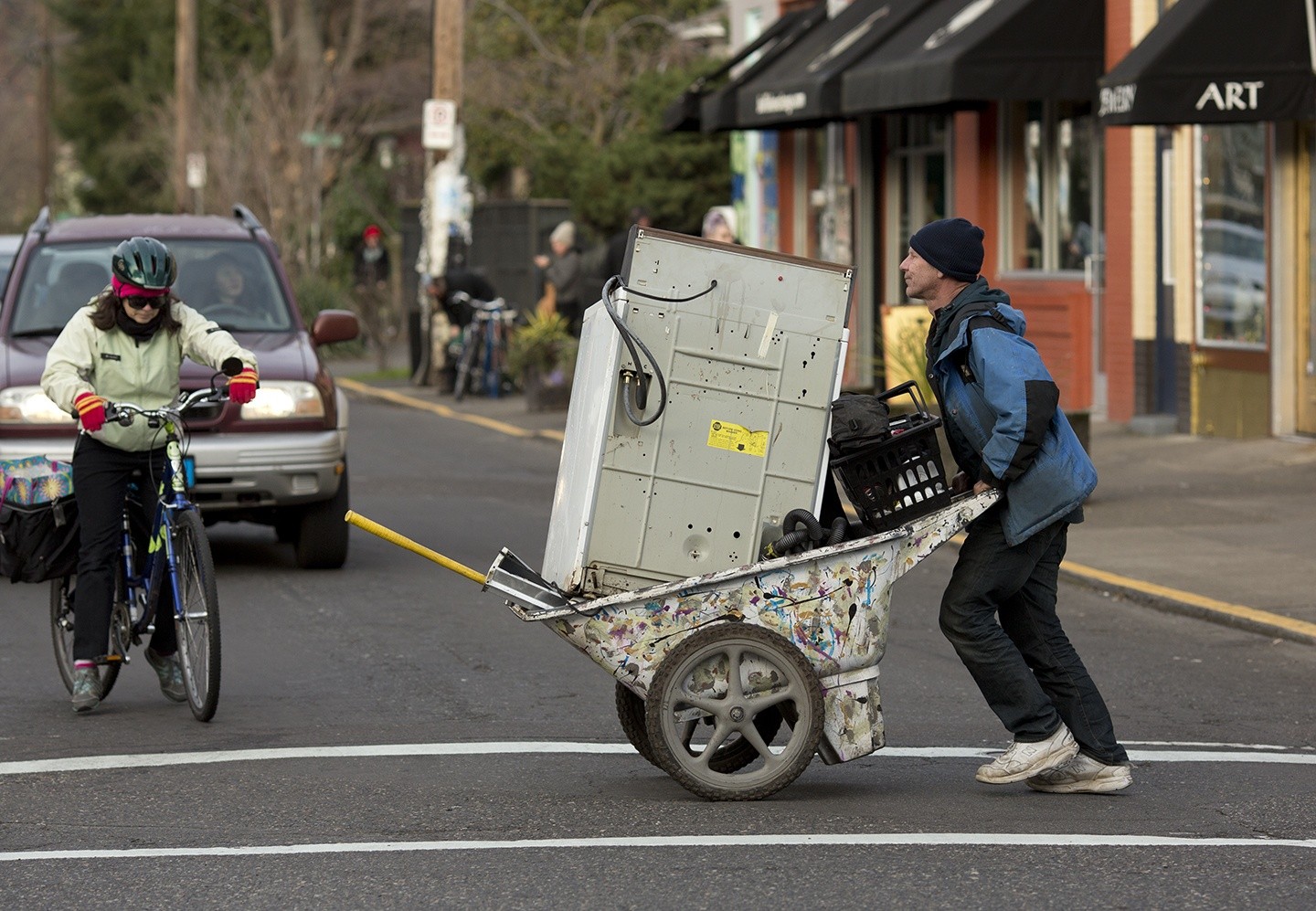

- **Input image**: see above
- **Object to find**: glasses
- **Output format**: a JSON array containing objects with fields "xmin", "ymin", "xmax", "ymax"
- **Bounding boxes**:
[{"xmin": 127, "ymin": 293, "xmax": 167, "ymax": 310}]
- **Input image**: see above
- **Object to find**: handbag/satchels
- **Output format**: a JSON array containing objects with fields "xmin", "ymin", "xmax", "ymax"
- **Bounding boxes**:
[{"xmin": 830, "ymin": 390, "xmax": 891, "ymax": 450}]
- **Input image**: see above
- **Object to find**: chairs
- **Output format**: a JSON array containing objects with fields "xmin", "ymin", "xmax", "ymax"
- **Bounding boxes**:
[{"xmin": 36, "ymin": 262, "xmax": 111, "ymax": 323}]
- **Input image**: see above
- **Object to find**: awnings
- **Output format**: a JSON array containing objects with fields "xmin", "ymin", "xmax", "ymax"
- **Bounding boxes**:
[
  {"xmin": 662, "ymin": 6, "xmax": 826, "ymax": 135},
  {"xmin": 734, "ymin": 0, "xmax": 931, "ymax": 129},
  {"xmin": 1096, "ymin": 0, "xmax": 1316, "ymax": 125},
  {"xmin": 839, "ymin": 0, "xmax": 1104, "ymax": 115}
]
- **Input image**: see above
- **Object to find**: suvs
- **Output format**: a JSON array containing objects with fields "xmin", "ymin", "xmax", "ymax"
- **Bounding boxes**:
[{"xmin": 0, "ymin": 203, "xmax": 360, "ymax": 577}]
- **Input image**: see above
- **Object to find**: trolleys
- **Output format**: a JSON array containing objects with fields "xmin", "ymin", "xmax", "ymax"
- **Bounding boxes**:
[{"xmin": 502, "ymin": 487, "xmax": 1005, "ymax": 804}]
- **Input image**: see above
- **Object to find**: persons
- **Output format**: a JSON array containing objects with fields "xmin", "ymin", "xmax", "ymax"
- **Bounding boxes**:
[
  {"xmin": 198, "ymin": 252, "xmax": 274, "ymax": 324},
  {"xmin": 41, "ymin": 236, "xmax": 260, "ymax": 711},
  {"xmin": 899, "ymin": 217, "xmax": 1133, "ymax": 795},
  {"xmin": 701, "ymin": 204, "xmax": 738, "ymax": 244},
  {"xmin": 427, "ymin": 274, "xmax": 496, "ymax": 399},
  {"xmin": 353, "ymin": 223, "xmax": 389, "ymax": 372},
  {"xmin": 532, "ymin": 219, "xmax": 586, "ymax": 338},
  {"xmin": 608, "ymin": 208, "xmax": 654, "ymax": 277}
]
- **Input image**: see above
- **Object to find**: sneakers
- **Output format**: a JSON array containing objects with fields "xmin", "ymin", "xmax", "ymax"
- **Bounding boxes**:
[
  {"xmin": 975, "ymin": 720, "xmax": 1080, "ymax": 784},
  {"xmin": 70, "ymin": 665, "xmax": 103, "ymax": 713},
  {"xmin": 143, "ymin": 647, "xmax": 192, "ymax": 703},
  {"xmin": 1029, "ymin": 748, "xmax": 1135, "ymax": 795}
]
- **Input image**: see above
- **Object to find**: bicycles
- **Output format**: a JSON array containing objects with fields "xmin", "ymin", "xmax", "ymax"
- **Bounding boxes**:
[
  {"xmin": 448, "ymin": 292, "xmax": 522, "ymax": 400},
  {"xmin": 47, "ymin": 356, "xmax": 262, "ymax": 725}
]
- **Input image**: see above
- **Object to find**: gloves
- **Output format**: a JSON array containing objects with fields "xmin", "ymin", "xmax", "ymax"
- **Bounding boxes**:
[
  {"xmin": 73, "ymin": 391, "xmax": 110, "ymax": 434},
  {"xmin": 228, "ymin": 367, "xmax": 259, "ymax": 404}
]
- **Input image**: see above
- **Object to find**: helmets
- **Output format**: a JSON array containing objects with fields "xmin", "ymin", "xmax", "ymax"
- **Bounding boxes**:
[{"xmin": 112, "ymin": 235, "xmax": 179, "ymax": 291}]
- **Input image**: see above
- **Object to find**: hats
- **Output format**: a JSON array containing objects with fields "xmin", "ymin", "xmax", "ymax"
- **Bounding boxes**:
[
  {"xmin": 549, "ymin": 220, "xmax": 578, "ymax": 246},
  {"xmin": 909, "ymin": 216, "xmax": 984, "ymax": 282}
]
